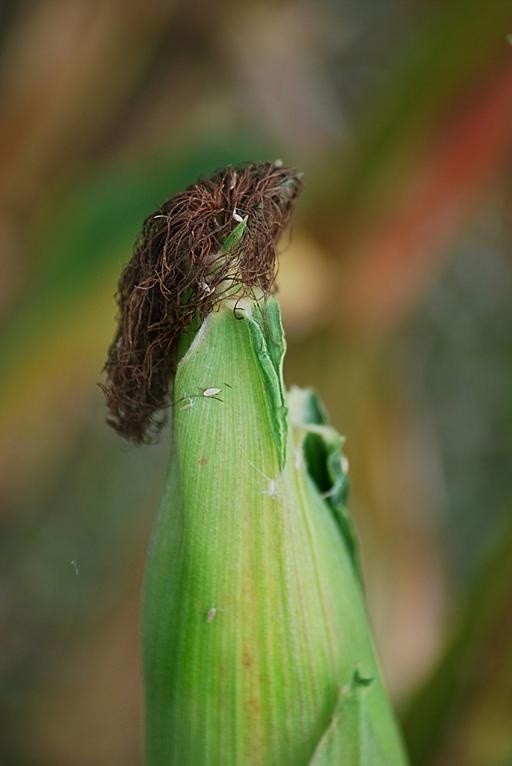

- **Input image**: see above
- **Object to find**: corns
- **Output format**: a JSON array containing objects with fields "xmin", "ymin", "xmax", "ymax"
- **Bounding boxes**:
[{"xmin": 100, "ymin": 158, "xmax": 407, "ymax": 766}]
